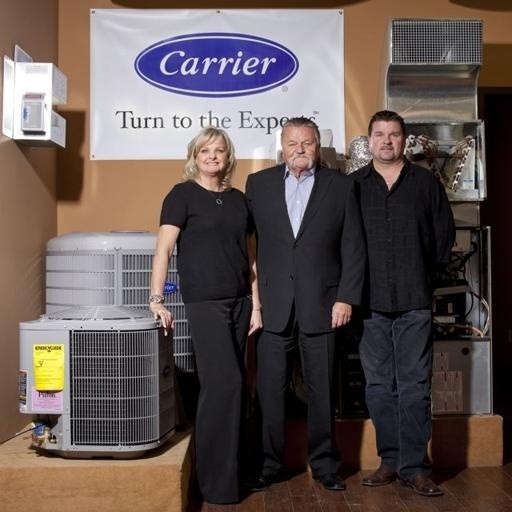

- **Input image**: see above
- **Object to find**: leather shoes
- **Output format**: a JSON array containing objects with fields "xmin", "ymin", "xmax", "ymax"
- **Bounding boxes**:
[
  {"xmin": 312, "ymin": 471, "xmax": 345, "ymax": 491},
  {"xmin": 406, "ymin": 472, "xmax": 442, "ymax": 496},
  {"xmin": 245, "ymin": 470, "xmax": 280, "ymax": 491},
  {"xmin": 362, "ymin": 464, "xmax": 398, "ymax": 486}
]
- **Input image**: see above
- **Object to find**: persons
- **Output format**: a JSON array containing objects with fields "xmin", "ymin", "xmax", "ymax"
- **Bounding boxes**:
[
  {"xmin": 347, "ymin": 109, "xmax": 457, "ymax": 497},
  {"xmin": 148, "ymin": 127, "xmax": 264, "ymax": 505},
  {"xmin": 244, "ymin": 117, "xmax": 368, "ymax": 491}
]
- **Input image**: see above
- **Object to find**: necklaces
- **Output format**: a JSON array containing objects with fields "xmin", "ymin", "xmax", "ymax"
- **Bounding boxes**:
[{"xmin": 205, "ymin": 185, "xmax": 226, "ymax": 206}]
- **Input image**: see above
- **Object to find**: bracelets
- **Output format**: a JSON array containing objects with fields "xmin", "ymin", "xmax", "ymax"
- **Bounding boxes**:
[
  {"xmin": 252, "ymin": 308, "xmax": 261, "ymax": 312},
  {"xmin": 149, "ymin": 294, "xmax": 165, "ymax": 305}
]
